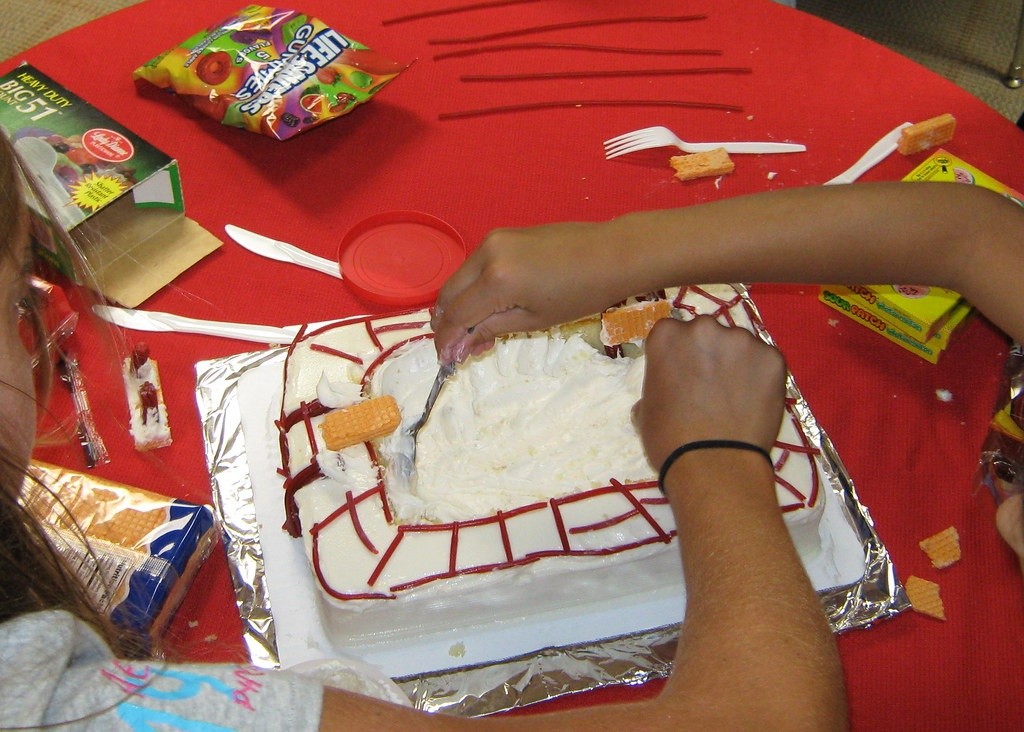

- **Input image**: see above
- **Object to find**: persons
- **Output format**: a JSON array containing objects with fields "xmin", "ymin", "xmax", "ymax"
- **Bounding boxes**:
[
  {"xmin": 0, "ymin": 127, "xmax": 849, "ymax": 732},
  {"xmin": 429, "ymin": 181, "xmax": 1024, "ymax": 570}
]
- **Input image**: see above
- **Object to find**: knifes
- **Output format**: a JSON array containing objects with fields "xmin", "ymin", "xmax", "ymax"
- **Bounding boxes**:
[
  {"xmin": 223, "ymin": 222, "xmax": 342, "ymax": 281},
  {"xmin": 91, "ymin": 303, "xmax": 295, "ymax": 346}
]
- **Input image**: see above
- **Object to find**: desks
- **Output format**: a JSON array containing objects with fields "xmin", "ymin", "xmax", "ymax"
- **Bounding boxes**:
[{"xmin": 0, "ymin": 0, "xmax": 1024, "ymax": 732}]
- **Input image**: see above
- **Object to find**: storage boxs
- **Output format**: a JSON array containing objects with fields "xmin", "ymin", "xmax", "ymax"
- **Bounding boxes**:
[{"xmin": 0, "ymin": 64, "xmax": 224, "ymax": 308}]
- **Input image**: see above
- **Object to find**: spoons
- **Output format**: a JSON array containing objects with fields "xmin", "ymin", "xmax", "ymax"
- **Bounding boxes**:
[
  {"xmin": 401, "ymin": 325, "xmax": 474, "ymax": 477},
  {"xmin": 603, "ymin": 125, "xmax": 807, "ymax": 160},
  {"xmin": 51, "ymin": 324, "xmax": 96, "ymax": 468}
]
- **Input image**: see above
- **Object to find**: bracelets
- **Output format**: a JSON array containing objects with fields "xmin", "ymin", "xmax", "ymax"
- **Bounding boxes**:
[{"xmin": 659, "ymin": 440, "xmax": 776, "ymax": 494}]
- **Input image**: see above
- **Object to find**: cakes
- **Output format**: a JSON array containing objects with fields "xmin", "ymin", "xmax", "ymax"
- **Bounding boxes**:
[{"xmin": 273, "ymin": 281, "xmax": 824, "ymax": 646}]
